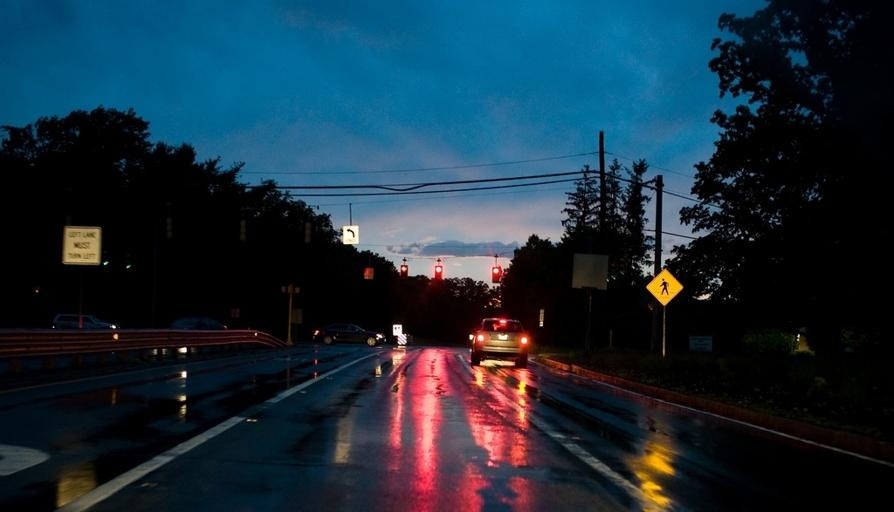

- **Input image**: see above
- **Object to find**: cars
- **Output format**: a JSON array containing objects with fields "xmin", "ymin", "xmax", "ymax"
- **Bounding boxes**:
[
  {"xmin": 470, "ymin": 318, "xmax": 528, "ymax": 367},
  {"xmin": 52, "ymin": 313, "xmax": 119, "ymax": 330},
  {"xmin": 312, "ymin": 322, "xmax": 386, "ymax": 346}
]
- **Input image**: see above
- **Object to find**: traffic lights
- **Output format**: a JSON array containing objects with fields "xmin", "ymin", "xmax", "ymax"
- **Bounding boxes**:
[
  {"xmin": 362, "ymin": 266, "xmax": 374, "ymax": 280},
  {"xmin": 400, "ymin": 266, "xmax": 409, "ymax": 278},
  {"xmin": 492, "ymin": 266, "xmax": 503, "ymax": 284},
  {"xmin": 434, "ymin": 265, "xmax": 443, "ymax": 280}
]
